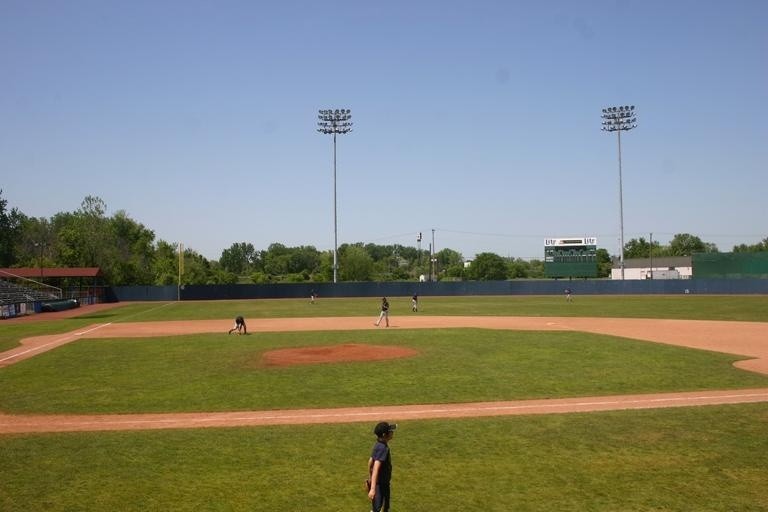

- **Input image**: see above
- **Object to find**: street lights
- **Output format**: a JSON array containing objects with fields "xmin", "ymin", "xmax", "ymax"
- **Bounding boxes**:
[
  {"xmin": 316, "ymin": 108, "xmax": 353, "ymax": 282},
  {"xmin": 600, "ymin": 105, "xmax": 638, "ymax": 281},
  {"xmin": 34, "ymin": 241, "xmax": 51, "ymax": 284}
]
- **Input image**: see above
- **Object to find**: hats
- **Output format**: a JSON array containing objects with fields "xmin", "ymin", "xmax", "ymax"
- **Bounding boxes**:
[{"xmin": 374, "ymin": 422, "xmax": 398, "ymax": 436}]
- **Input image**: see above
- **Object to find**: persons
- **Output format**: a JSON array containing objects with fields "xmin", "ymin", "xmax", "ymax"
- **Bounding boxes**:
[
  {"xmin": 374, "ymin": 297, "xmax": 390, "ymax": 327},
  {"xmin": 564, "ymin": 288, "xmax": 572, "ymax": 303},
  {"xmin": 309, "ymin": 289, "xmax": 315, "ymax": 304},
  {"xmin": 412, "ymin": 293, "xmax": 418, "ymax": 312},
  {"xmin": 228, "ymin": 316, "xmax": 246, "ymax": 335},
  {"xmin": 365, "ymin": 422, "xmax": 398, "ymax": 512}
]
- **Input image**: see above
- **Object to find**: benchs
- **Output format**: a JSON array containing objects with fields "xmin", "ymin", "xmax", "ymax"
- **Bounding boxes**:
[{"xmin": 0, "ymin": 276, "xmax": 59, "ymax": 320}]
